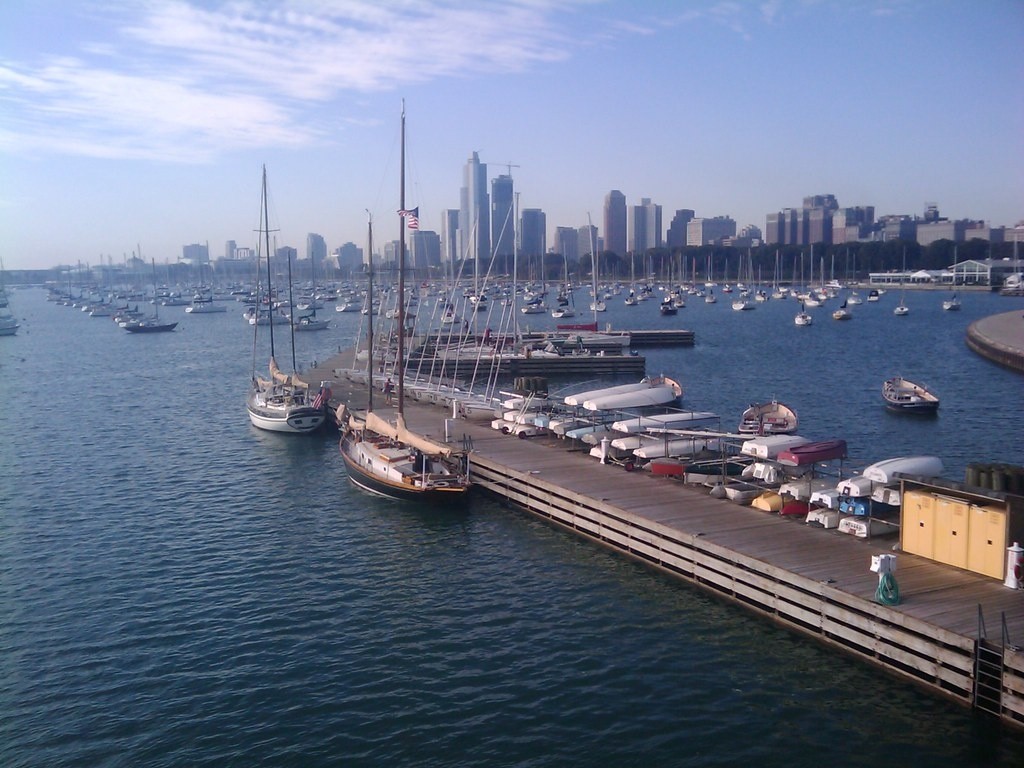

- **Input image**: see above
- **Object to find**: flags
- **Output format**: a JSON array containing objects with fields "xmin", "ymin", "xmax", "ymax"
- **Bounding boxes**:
[
  {"xmin": 398, "ymin": 206, "xmax": 419, "ymax": 229},
  {"xmin": 312, "ymin": 386, "xmax": 325, "ymax": 410}
]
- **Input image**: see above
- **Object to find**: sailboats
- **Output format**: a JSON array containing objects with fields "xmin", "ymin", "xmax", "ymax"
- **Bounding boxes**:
[
  {"xmin": 0, "ymin": 180, "xmax": 962, "ymax": 541},
  {"xmin": 333, "ymin": 94, "xmax": 476, "ymax": 502},
  {"xmin": 244, "ymin": 161, "xmax": 336, "ymax": 435}
]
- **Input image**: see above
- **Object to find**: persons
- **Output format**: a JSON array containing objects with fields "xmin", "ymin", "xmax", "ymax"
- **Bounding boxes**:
[
  {"xmin": 465, "ymin": 319, "xmax": 468, "ymax": 333},
  {"xmin": 485, "ymin": 328, "xmax": 492, "ymax": 345},
  {"xmin": 384, "ymin": 378, "xmax": 392, "ymax": 404}
]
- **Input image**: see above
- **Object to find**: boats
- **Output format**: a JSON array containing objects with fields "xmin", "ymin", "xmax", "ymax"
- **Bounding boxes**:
[
  {"xmin": 881, "ymin": 377, "xmax": 941, "ymax": 416},
  {"xmin": 736, "ymin": 393, "xmax": 800, "ymax": 437}
]
